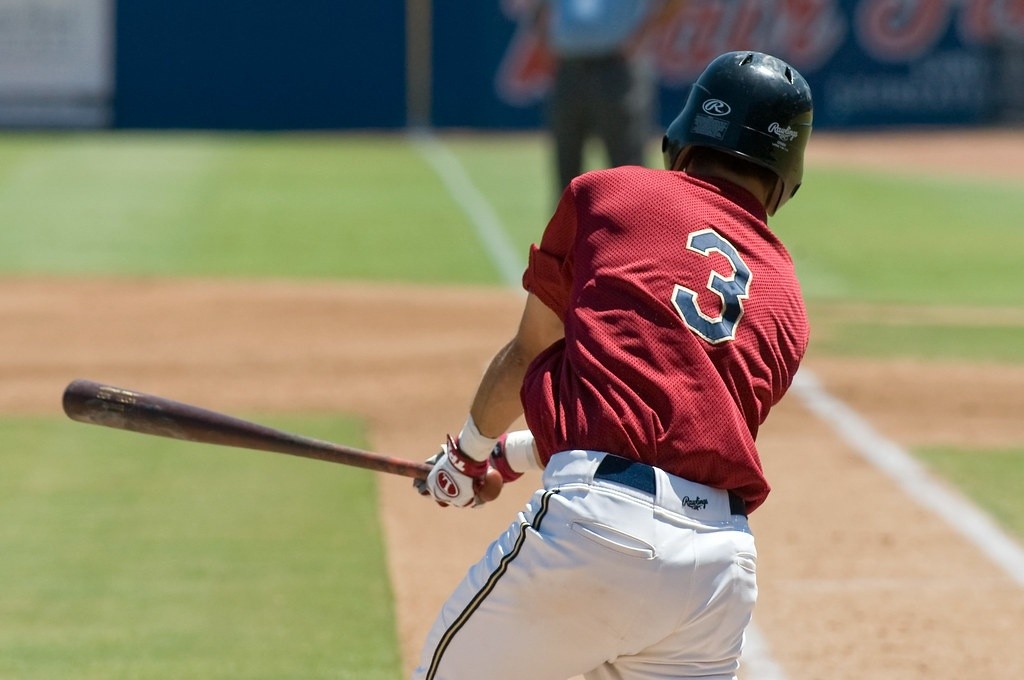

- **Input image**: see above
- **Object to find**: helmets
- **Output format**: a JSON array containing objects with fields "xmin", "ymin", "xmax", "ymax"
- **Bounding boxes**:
[{"xmin": 661, "ymin": 50, "xmax": 813, "ymax": 217}]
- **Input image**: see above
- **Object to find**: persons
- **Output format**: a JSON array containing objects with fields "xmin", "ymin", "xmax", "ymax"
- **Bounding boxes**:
[
  {"xmin": 532, "ymin": 0, "xmax": 648, "ymax": 202},
  {"xmin": 413, "ymin": 49, "xmax": 814, "ymax": 680}
]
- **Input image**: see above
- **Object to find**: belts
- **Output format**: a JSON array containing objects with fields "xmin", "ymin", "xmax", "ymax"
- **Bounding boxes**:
[{"xmin": 594, "ymin": 454, "xmax": 750, "ymax": 520}]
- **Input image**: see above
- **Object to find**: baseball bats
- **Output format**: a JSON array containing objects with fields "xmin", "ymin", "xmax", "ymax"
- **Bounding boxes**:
[{"xmin": 63, "ymin": 379, "xmax": 504, "ymax": 500}]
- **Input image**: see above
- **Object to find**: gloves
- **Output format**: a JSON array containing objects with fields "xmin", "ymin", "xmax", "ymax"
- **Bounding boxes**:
[
  {"xmin": 412, "ymin": 432, "xmax": 524, "ymax": 507},
  {"xmin": 424, "ymin": 434, "xmax": 491, "ymax": 509}
]
